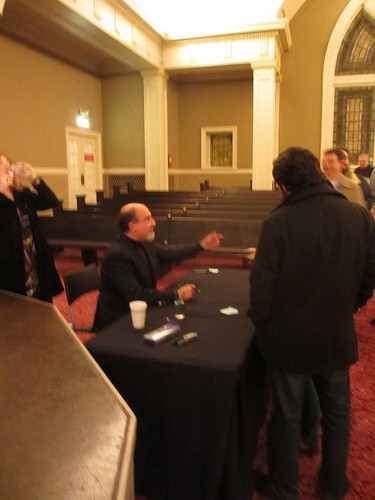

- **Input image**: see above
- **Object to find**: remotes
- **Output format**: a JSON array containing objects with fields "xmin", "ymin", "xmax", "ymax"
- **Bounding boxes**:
[{"xmin": 144, "ymin": 325, "xmax": 180, "ymax": 346}]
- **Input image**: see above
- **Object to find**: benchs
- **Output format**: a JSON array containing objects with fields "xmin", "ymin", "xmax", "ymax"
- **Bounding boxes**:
[{"xmin": 38, "ymin": 180, "xmax": 281, "ymax": 269}]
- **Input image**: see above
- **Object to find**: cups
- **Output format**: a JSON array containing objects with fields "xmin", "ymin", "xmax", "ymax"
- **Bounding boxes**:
[{"xmin": 129, "ymin": 300, "xmax": 147, "ymax": 329}]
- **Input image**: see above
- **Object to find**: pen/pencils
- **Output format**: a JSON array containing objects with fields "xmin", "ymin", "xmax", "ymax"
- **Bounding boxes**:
[{"xmin": 195, "ymin": 288, "xmax": 201, "ymax": 293}]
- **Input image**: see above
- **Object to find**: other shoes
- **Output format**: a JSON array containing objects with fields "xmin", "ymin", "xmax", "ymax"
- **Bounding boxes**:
[
  {"xmin": 328, "ymin": 479, "xmax": 349, "ymax": 496},
  {"xmin": 254, "ymin": 471, "xmax": 266, "ymax": 495},
  {"xmin": 302, "ymin": 437, "xmax": 319, "ymax": 455}
]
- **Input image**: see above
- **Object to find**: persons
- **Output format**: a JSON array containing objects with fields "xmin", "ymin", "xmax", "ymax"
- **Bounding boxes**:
[
  {"xmin": 300, "ymin": 375, "xmax": 323, "ymax": 457},
  {"xmin": 0, "ymin": 152, "xmax": 65, "ymax": 305},
  {"xmin": 247, "ymin": 146, "xmax": 375, "ymax": 500},
  {"xmin": 321, "ymin": 148, "xmax": 375, "ymax": 211},
  {"xmin": 90, "ymin": 202, "xmax": 225, "ymax": 334}
]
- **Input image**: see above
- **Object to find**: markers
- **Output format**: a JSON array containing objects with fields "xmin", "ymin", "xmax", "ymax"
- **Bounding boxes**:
[{"xmin": 193, "ymin": 270, "xmax": 209, "ymax": 273}]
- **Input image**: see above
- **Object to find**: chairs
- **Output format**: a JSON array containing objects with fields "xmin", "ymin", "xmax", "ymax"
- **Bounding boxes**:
[{"xmin": 62, "ymin": 264, "xmax": 100, "ymax": 332}]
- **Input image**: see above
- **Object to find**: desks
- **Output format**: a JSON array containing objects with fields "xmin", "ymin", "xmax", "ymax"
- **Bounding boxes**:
[{"xmin": 84, "ymin": 266, "xmax": 272, "ymax": 500}]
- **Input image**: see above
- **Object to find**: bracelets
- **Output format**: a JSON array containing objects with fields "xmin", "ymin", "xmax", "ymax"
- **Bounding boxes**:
[{"xmin": 32, "ymin": 176, "xmax": 38, "ymax": 184}]
